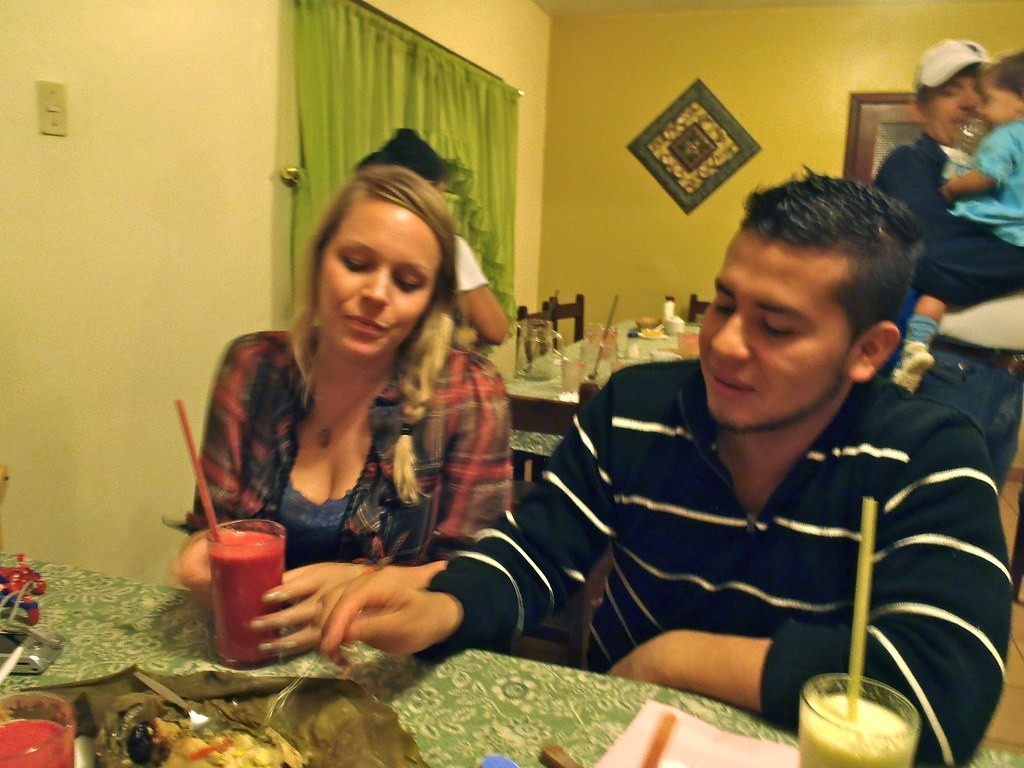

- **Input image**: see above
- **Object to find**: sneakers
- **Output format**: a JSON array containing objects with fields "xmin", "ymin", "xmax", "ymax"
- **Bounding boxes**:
[{"xmin": 890, "ymin": 343, "xmax": 935, "ymax": 394}]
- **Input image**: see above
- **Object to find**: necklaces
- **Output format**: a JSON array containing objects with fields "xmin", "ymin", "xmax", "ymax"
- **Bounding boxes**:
[{"xmin": 315, "ymin": 377, "xmax": 380, "ymax": 446}]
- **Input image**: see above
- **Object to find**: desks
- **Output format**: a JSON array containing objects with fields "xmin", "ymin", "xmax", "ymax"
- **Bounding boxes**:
[
  {"xmin": 499, "ymin": 318, "xmax": 705, "ymax": 456},
  {"xmin": 0, "ymin": 555, "xmax": 804, "ymax": 766}
]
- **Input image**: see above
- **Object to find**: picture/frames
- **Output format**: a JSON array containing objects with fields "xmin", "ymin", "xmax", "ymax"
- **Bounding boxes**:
[{"xmin": 627, "ymin": 79, "xmax": 761, "ymax": 216}]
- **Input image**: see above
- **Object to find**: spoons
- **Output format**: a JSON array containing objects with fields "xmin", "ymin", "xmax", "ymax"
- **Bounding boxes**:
[
  {"xmin": 265, "ymin": 593, "xmax": 367, "ymax": 722},
  {"xmin": 133, "ymin": 672, "xmax": 231, "ymax": 736}
]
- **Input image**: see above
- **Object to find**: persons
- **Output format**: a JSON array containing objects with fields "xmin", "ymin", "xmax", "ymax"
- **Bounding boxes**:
[
  {"xmin": 875, "ymin": 40, "xmax": 1024, "ymax": 489},
  {"xmin": 180, "ymin": 167, "xmax": 514, "ymax": 654},
  {"xmin": 360, "ymin": 128, "xmax": 507, "ymax": 345},
  {"xmin": 320, "ymin": 177, "xmax": 1013, "ymax": 768},
  {"xmin": 891, "ymin": 51, "xmax": 1023, "ymax": 387}
]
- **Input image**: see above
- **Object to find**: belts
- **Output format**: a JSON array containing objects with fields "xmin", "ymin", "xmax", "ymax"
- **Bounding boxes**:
[{"xmin": 930, "ymin": 339, "xmax": 1024, "ymax": 378}]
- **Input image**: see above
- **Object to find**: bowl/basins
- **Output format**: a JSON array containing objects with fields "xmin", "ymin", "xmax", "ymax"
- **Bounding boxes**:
[{"xmin": 635, "ymin": 316, "xmax": 661, "ymax": 330}]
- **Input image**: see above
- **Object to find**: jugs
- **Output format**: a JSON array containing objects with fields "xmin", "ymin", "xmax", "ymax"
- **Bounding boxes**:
[{"xmin": 516, "ymin": 317, "xmax": 564, "ymax": 380}]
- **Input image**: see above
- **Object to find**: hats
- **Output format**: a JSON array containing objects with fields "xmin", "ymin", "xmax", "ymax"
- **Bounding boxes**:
[
  {"xmin": 356, "ymin": 129, "xmax": 447, "ymax": 186},
  {"xmin": 911, "ymin": 38, "xmax": 997, "ymax": 90}
]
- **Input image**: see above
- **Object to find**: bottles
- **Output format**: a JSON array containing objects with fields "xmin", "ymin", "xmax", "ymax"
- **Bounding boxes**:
[
  {"xmin": 662, "ymin": 296, "xmax": 675, "ymax": 323},
  {"xmin": 627, "ymin": 333, "xmax": 638, "ymax": 360},
  {"xmin": 937, "ymin": 114, "xmax": 988, "ymax": 182}
]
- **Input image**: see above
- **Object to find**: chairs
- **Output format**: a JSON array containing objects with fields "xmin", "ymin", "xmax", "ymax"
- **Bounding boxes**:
[{"xmin": 503, "ymin": 291, "xmax": 710, "ymax": 671}]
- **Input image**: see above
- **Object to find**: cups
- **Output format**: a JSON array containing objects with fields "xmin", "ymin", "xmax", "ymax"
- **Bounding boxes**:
[
  {"xmin": 578, "ymin": 322, "xmax": 617, "ymax": 386},
  {"xmin": 677, "ymin": 322, "xmax": 700, "ymax": 350},
  {"xmin": 664, "ymin": 316, "xmax": 685, "ymax": 334},
  {"xmin": 207, "ymin": 519, "xmax": 285, "ymax": 671},
  {"xmin": 650, "ymin": 352, "xmax": 682, "ymax": 361},
  {"xmin": 0, "ymin": 694, "xmax": 75, "ymax": 768},
  {"xmin": 799, "ymin": 673, "xmax": 920, "ymax": 768}
]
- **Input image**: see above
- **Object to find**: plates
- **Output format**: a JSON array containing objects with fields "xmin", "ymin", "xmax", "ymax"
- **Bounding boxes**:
[
  {"xmin": 19, "ymin": 664, "xmax": 430, "ymax": 768},
  {"xmin": 637, "ymin": 332, "xmax": 666, "ymax": 339}
]
